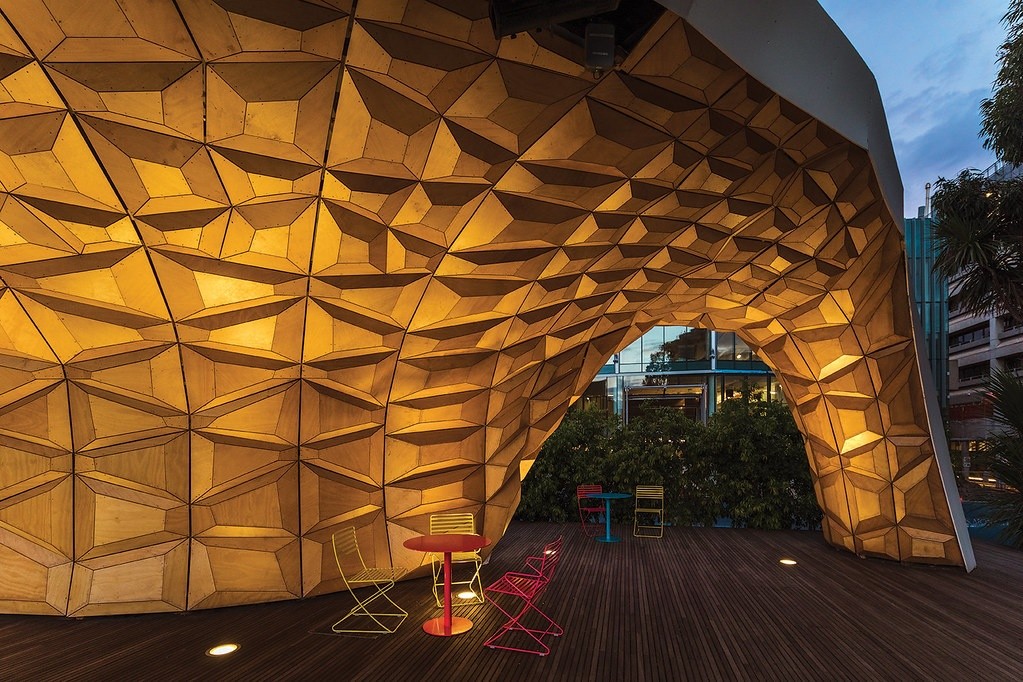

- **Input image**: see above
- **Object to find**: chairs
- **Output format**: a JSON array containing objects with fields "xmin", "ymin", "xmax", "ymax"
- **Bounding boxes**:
[
  {"xmin": 484, "ymin": 534, "xmax": 563, "ymax": 656},
  {"xmin": 577, "ymin": 485, "xmax": 606, "ymax": 537},
  {"xmin": 633, "ymin": 485, "xmax": 664, "ymax": 539},
  {"xmin": 430, "ymin": 513, "xmax": 485, "ymax": 607},
  {"xmin": 332, "ymin": 526, "xmax": 408, "ymax": 633}
]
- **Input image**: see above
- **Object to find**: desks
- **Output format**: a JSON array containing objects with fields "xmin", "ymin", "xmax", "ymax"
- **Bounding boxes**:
[
  {"xmin": 403, "ymin": 534, "xmax": 492, "ymax": 637},
  {"xmin": 587, "ymin": 493, "xmax": 631, "ymax": 543}
]
